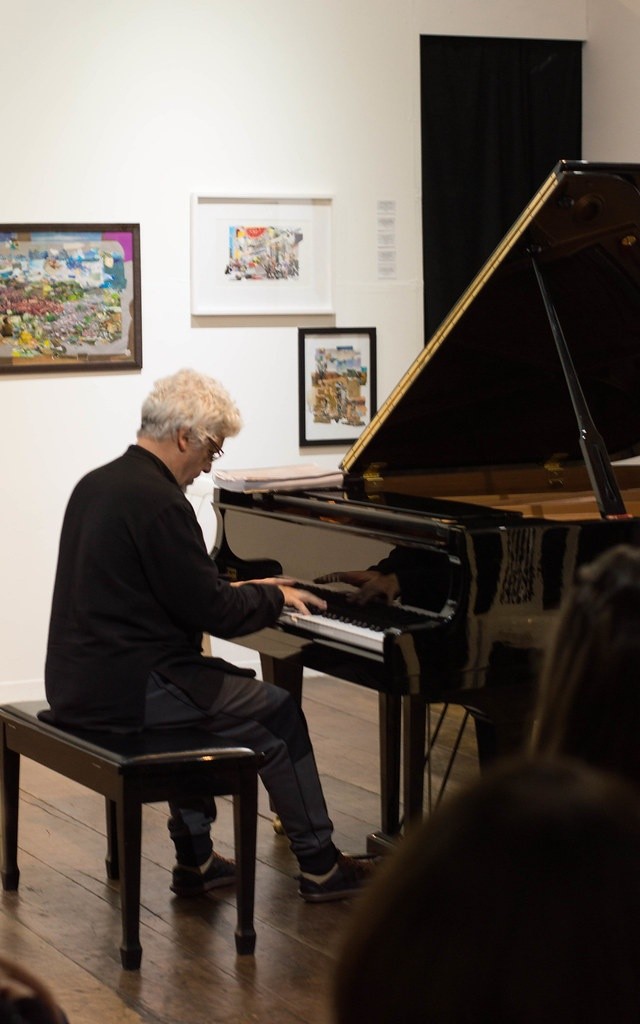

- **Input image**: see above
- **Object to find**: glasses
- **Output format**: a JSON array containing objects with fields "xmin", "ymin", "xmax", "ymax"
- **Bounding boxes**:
[{"xmin": 176, "ymin": 427, "xmax": 224, "ymax": 463}]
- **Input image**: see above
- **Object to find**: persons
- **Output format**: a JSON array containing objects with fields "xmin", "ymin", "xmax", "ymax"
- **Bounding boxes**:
[
  {"xmin": 44, "ymin": 366, "xmax": 386, "ymax": 904},
  {"xmin": 312, "ymin": 541, "xmax": 460, "ymax": 614},
  {"xmin": 322, "ymin": 544, "xmax": 640, "ymax": 1024}
]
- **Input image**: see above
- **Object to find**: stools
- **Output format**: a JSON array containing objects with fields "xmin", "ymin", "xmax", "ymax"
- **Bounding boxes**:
[{"xmin": 0, "ymin": 700, "xmax": 258, "ymax": 971}]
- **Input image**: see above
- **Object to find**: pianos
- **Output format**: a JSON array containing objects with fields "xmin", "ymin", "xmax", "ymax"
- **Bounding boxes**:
[{"xmin": 193, "ymin": 157, "xmax": 640, "ymax": 862}]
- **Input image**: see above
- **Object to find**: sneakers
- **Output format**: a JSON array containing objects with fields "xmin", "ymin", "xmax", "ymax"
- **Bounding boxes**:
[
  {"xmin": 293, "ymin": 856, "xmax": 382, "ymax": 901},
  {"xmin": 170, "ymin": 849, "xmax": 237, "ymax": 897}
]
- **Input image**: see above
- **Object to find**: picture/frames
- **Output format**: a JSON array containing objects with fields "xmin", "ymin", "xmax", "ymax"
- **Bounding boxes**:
[
  {"xmin": 0, "ymin": 223, "xmax": 143, "ymax": 375},
  {"xmin": 297, "ymin": 327, "xmax": 378, "ymax": 447},
  {"xmin": 189, "ymin": 190, "xmax": 336, "ymax": 318}
]
